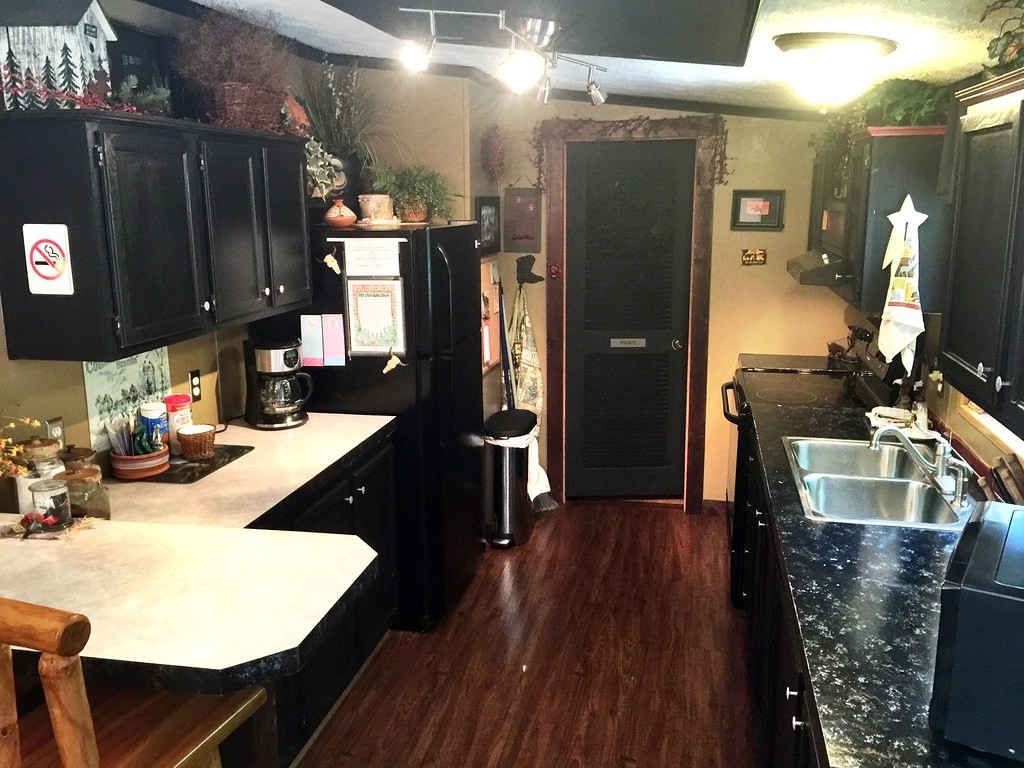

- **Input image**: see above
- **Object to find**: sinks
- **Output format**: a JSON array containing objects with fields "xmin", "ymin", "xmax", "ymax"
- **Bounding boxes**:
[
  {"xmin": 781, "ymin": 436, "xmax": 934, "ymax": 479},
  {"xmin": 794, "ymin": 478, "xmax": 975, "ymax": 531}
]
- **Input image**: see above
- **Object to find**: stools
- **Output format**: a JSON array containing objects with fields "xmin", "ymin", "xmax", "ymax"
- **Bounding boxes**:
[{"xmin": 0, "ymin": 595, "xmax": 267, "ymax": 768}]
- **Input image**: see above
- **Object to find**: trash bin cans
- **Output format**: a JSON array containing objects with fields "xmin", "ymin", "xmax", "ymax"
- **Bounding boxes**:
[{"xmin": 484, "ymin": 409, "xmax": 539, "ymax": 550}]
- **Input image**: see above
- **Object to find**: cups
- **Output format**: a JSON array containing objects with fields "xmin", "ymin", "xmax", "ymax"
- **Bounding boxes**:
[
  {"xmin": 358, "ymin": 195, "xmax": 390, "ymax": 220},
  {"xmin": 14, "ymin": 434, "xmax": 109, "ymax": 533}
]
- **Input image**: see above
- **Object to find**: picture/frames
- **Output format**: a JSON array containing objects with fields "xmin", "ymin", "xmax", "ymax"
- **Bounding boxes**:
[
  {"xmin": 729, "ymin": 189, "xmax": 786, "ymax": 232},
  {"xmin": 475, "ymin": 196, "xmax": 501, "ymax": 256}
]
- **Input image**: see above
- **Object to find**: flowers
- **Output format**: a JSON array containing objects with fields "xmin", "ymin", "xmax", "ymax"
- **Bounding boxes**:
[
  {"xmin": 292, "ymin": 46, "xmax": 409, "ymax": 173},
  {"xmin": 0, "ymin": 415, "xmax": 41, "ymax": 479}
]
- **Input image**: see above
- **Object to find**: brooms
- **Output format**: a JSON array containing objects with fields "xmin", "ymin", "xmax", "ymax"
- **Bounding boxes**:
[{"xmin": 501, "ymin": 293, "xmax": 559, "ymax": 512}]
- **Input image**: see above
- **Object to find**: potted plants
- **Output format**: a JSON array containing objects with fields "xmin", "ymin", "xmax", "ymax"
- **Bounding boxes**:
[
  {"xmin": 822, "ymin": 78, "xmax": 947, "ymax": 149},
  {"xmin": 367, "ymin": 164, "xmax": 466, "ymax": 224}
]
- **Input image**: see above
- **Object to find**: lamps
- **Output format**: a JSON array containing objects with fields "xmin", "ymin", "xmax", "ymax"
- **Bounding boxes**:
[
  {"xmin": 772, "ymin": 32, "xmax": 896, "ymax": 111},
  {"xmin": 397, "ymin": 4, "xmax": 609, "ymax": 107}
]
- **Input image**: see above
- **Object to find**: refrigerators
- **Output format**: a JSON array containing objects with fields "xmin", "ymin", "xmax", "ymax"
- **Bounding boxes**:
[{"xmin": 249, "ymin": 216, "xmax": 488, "ymax": 633}]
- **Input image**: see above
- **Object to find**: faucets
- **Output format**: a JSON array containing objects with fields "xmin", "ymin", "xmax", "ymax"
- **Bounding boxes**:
[
  {"xmin": 947, "ymin": 457, "xmax": 970, "ymax": 508},
  {"xmin": 868, "ymin": 424, "xmax": 951, "ymax": 476}
]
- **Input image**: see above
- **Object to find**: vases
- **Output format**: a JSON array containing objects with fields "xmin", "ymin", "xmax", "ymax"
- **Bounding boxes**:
[
  {"xmin": 359, "ymin": 194, "xmax": 395, "ymax": 223},
  {"xmin": 323, "ymin": 198, "xmax": 357, "ymax": 227},
  {"xmin": 327, "ymin": 148, "xmax": 365, "ymax": 218}
]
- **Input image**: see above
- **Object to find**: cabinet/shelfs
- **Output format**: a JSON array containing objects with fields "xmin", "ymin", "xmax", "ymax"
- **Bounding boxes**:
[
  {"xmin": 937, "ymin": 98, "xmax": 1024, "ymax": 442},
  {"xmin": 0, "ymin": 106, "xmax": 216, "ymax": 364},
  {"xmin": 807, "ymin": 135, "xmax": 951, "ymax": 315},
  {"xmin": 203, "ymin": 123, "xmax": 314, "ymax": 336},
  {"xmin": 261, "ymin": 423, "xmax": 400, "ymax": 768},
  {"xmin": 727, "ymin": 401, "xmax": 818, "ymax": 768}
]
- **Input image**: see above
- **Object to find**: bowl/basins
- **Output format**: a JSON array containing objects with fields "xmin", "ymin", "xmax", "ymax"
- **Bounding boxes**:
[{"xmin": 110, "ymin": 443, "xmax": 169, "ymax": 478}]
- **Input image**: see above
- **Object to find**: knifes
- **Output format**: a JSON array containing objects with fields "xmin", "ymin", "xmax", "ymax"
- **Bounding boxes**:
[{"xmin": 977, "ymin": 451, "xmax": 1024, "ymax": 504}]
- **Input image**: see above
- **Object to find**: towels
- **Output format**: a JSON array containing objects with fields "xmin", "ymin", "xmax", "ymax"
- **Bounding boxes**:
[{"xmin": 878, "ymin": 238, "xmax": 926, "ymax": 377}]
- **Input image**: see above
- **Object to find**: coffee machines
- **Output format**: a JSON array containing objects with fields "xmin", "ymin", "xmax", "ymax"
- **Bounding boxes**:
[{"xmin": 243, "ymin": 335, "xmax": 312, "ymax": 429}]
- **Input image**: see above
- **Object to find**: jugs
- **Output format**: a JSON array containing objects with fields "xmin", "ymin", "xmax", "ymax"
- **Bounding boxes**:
[{"xmin": 261, "ymin": 371, "xmax": 312, "ymax": 413}]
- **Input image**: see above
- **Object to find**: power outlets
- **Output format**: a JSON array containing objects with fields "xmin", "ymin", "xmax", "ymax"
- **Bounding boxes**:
[
  {"xmin": 189, "ymin": 369, "xmax": 202, "ymax": 403},
  {"xmin": 46, "ymin": 416, "xmax": 67, "ymax": 451},
  {"xmin": 937, "ymin": 380, "xmax": 944, "ymax": 398}
]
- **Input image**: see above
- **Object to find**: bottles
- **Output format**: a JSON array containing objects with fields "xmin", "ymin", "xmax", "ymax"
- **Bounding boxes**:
[
  {"xmin": 141, "ymin": 395, "xmax": 195, "ymax": 455},
  {"xmin": 324, "ymin": 199, "xmax": 356, "ymax": 226}
]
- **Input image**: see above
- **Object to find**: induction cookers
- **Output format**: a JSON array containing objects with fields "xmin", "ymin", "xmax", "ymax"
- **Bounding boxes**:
[{"xmin": 738, "ymin": 323, "xmax": 894, "ymax": 407}]
